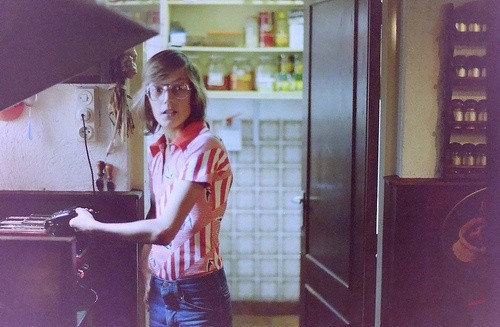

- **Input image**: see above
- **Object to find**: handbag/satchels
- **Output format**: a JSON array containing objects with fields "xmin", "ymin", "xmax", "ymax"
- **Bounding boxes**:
[{"xmin": 44, "ymin": 207, "xmax": 99, "ymax": 237}]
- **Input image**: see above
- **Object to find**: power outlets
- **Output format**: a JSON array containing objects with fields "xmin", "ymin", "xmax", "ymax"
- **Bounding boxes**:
[{"xmin": 73, "ymin": 85, "xmax": 101, "ymax": 143}]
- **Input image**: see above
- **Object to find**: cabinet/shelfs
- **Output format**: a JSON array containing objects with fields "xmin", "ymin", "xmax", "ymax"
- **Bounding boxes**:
[
  {"xmin": 382, "ymin": 172, "xmax": 500, "ymax": 327},
  {"xmin": 159, "ymin": 0, "xmax": 304, "ymax": 100},
  {"xmin": 440, "ymin": 2, "xmax": 489, "ymax": 179}
]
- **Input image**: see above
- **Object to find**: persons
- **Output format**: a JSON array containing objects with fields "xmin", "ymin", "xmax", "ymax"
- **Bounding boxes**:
[{"xmin": 69, "ymin": 49, "xmax": 233, "ymax": 327}]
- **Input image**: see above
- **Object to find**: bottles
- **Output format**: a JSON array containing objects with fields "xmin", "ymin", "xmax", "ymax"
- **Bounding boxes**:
[
  {"xmin": 245, "ymin": 7, "xmax": 304, "ymax": 49},
  {"xmin": 188, "ymin": 53, "xmax": 303, "ymax": 91}
]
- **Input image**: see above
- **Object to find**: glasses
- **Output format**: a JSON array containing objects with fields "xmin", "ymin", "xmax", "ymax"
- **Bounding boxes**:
[{"xmin": 147, "ymin": 84, "xmax": 191, "ymax": 101}]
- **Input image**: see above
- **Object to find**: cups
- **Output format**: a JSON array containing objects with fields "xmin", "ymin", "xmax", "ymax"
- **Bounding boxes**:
[{"xmin": 448, "ymin": 17, "xmax": 487, "ymax": 167}]
